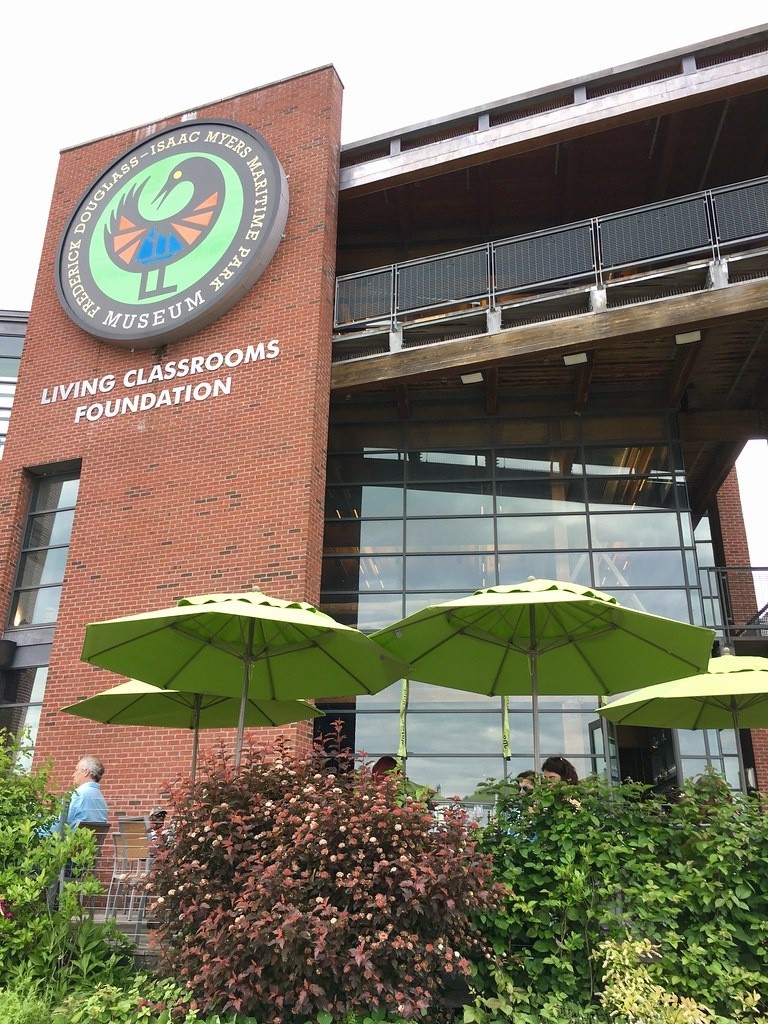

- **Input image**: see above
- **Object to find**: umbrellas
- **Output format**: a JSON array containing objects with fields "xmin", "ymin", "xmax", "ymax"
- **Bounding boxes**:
[
  {"xmin": 367, "ymin": 576, "xmax": 716, "ymax": 801},
  {"xmin": 80, "ymin": 586, "xmax": 418, "ymax": 781},
  {"xmin": 594, "ymin": 647, "xmax": 768, "ymax": 812},
  {"xmin": 59, "ymin": 681, "xmax": 326, "ymax": 806}
]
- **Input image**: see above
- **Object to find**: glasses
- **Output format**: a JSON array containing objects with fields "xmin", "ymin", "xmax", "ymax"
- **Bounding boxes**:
[
  {"xmin": 518, "ymin": 785, "xmax": 529, "ymax": 792},
  {"xmin": 155, "ymin": 813, "xmax": 165, "ymax": 817}
]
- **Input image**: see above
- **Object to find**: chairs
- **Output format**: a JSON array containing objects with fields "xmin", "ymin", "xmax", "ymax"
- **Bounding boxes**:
[{"xmin": 27, "ymin": 815, "xmax": 177, "ymax": 948}]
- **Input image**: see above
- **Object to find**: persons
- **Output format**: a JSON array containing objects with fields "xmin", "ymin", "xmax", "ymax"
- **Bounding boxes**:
[
  {"xmin": 34, "ymin": 757, "xmax": 109, "ymax": 909},
  {"xmin": 541, "ymin": 756, "xmax": 583, "ymax": 814},
  {"xmin": 370, "ymin": 756, "xmax": 400, "ymax": 782},
  {"xmin": 696, "ymin": 774, "xmax": 733, "ymax": 824},
  {"xmin": 500, "ymin": 770, "xmax": 539, "ymax": 842}
]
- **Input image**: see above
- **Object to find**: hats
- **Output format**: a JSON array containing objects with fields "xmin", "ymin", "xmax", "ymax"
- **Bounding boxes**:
[{"xmin": 148, "ymin": 807, "xmax": 167, "ymax": 817}]
